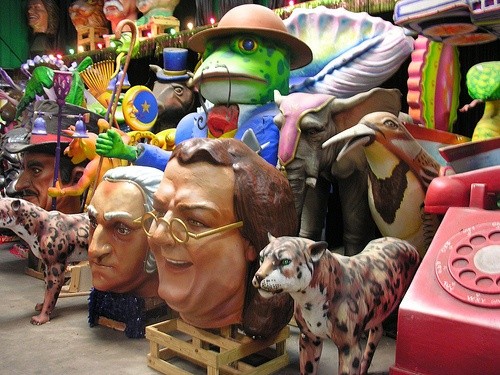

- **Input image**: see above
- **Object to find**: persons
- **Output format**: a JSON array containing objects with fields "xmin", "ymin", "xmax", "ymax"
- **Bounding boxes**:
[
  {"xmin": 104, "ymin": 0, "xmax": 138, "ymax": 32},
  {"xmin": 5, "ymin": 100, "xmax": 104, "ymax": 213},
  {"xmin": 88, "ymin": 166, "xmax": 163, "ymax": 297},
  {"xmin": 27, "ymin": 0, "xmax": 59, "ymax": 52},
  {"xmin": 147, "ymin": 137, "xmax": 299, "ymax": 340}
]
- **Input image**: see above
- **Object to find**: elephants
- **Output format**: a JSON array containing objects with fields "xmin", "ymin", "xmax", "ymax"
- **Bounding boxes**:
[{"xmin": 273, "ymin": 87, "xmax": 404, "ymax": 256}]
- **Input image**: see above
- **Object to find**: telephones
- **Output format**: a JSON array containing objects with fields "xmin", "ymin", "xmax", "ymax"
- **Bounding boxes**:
[{"xmin": 389, "ymin": 164, "xmax": 500, "ymax": 375}]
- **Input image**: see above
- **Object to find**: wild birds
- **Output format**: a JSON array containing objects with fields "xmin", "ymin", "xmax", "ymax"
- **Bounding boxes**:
[{"xmin": 321, "ymin": 110, "xmax": 457, "ymax": 262}]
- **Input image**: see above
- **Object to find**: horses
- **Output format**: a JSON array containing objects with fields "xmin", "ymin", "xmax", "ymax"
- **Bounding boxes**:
[{"xmin": 148, "ymin": 63, "xmax": 200, "ymax": 134}]
